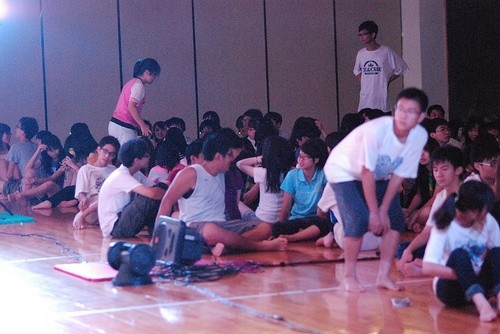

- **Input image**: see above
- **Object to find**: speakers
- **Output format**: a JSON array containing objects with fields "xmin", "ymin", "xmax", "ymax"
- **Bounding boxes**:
[{"xmin": 150, "ymin": 215, "xmax": 204, "ymax": 268}]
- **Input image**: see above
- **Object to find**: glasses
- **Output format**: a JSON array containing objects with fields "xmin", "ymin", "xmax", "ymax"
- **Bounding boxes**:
[
  {"xmin": 297, "ymin": 155, "xmax": 314, "ymax": 160},
  {"xmin": 101, "ymin": 147, "xmax": 117, "ymax": 158},
  {"xmin": 16, "ymin": 126, "xmax": 23, "ymax": 130},
  {"xmin": 393, "ymin": 104, "xmax": 421, "ymax": 116},
  {"xmin": 358, "ymin": 32, "xmax": 371, "ymax": 37}
]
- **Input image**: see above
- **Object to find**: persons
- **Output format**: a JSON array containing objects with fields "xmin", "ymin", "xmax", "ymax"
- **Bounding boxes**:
[
  {"xmin": 0, "ymin": 103, "xmax": 500, "ymax": 278},
  {"xmin": 421, "ymin": 179, "xmax": 500, "ymax": 323},
  {"xmin": 108, "ymin": 57, "xmax": 162, "ymax": 167},
  {"xmin": 353, "ymin": 20, "xmax": 410, "ymax": 116},
  {"xmin": 323, "ymin": 87, "xmax": 428, "ymax": 292}
]
